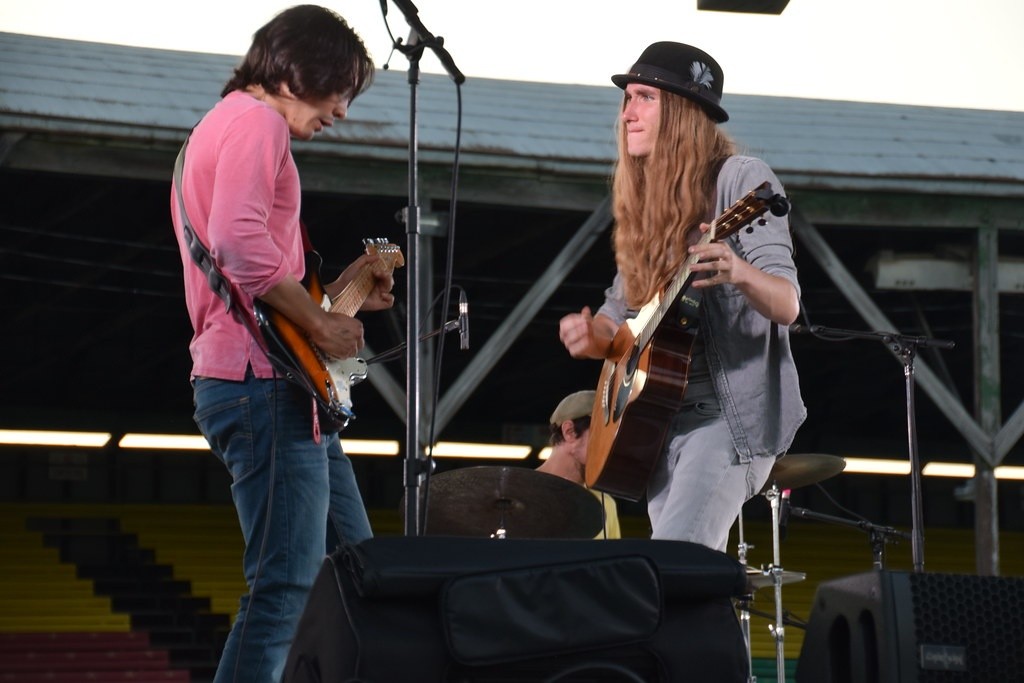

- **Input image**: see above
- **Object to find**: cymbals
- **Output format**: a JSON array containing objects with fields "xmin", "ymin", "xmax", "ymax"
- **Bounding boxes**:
[
  {"xmin": 759, "ymin": 453, "xmax": 846, "ymax": 491},
  {"xmin": 403, "ymin": 465, "xmax": 607, "ymax": 542},
  {"xmin": 744, "ymin": 566, "xmax": 806, "ymax": 587}
]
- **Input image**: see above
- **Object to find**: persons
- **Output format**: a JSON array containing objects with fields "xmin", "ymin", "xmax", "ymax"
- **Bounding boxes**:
[
  {"xmin": 530, "ymin": 388, "xmax": 620, "ymax": 539},
  {"xmin": 557, "ymin": 35, "xmax": 810, "ymax": 557},
  {"xmin": 166, "ymin": 5, "xmax": 395, "ymax": 682}
]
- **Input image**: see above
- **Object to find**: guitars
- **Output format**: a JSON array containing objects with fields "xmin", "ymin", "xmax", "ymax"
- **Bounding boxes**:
[
  {"xmin": 584, "ymin": 182, "xmax": 791, "ymax": 503},
  {"xmin": 253, "ymin": 236, "xmax": 405, "ymax": 436}
]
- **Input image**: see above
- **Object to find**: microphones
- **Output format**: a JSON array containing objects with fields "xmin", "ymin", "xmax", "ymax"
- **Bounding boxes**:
[
  {"xmin": 457, "ymin": 290, "xmax": 469, "ymax": 350},
  {"xmin": 779, "ymin": 497, "xmax": 791, "ymax": 538}
]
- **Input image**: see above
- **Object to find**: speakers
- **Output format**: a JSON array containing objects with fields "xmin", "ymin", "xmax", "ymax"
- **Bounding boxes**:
[
  {"xmin": 793, "ymin": 569, "xmax": 1024, "ymax": 683},
  {"xmin": 280, "ymin": 532, "xmax": 750, "ymax": 683}
]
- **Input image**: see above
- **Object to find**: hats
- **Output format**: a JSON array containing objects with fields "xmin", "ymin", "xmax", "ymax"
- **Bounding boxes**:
[
  {"xmin": 549, "ymin": 390, "xmax": 597, "ymax": 431},
  {"xmin": 611, "ymin": 42, "xmax": 728, "ymax": 120}
]
